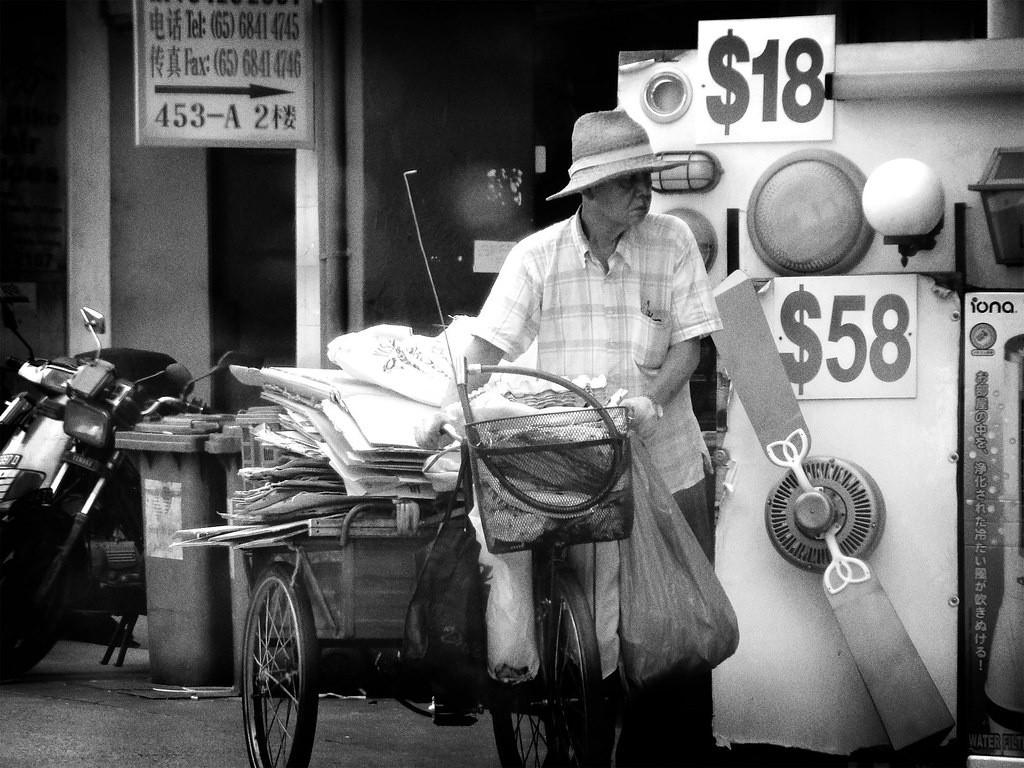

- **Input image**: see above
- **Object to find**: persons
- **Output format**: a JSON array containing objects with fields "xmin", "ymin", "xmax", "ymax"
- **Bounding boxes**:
[{"xmin": 415, "ymin": 109, "xmax": 723, "ymax": 768}]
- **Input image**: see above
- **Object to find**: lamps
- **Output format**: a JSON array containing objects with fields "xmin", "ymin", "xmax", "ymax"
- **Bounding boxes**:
[{"xmin": 864, "ymin": 157, "xmax": 947, "ymax": 269}]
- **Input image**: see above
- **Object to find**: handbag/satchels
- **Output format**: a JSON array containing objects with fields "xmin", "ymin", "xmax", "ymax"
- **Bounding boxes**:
[
  {"xmin": 562, "ymin": 430, "xmax": 740, "ymax": 688},
  {"xmin": 402, "ymin": 436, "xmax": 540, "ymax": 687}
]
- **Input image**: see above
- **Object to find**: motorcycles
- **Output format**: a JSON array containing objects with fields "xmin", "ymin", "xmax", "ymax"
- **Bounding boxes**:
[{"xmin": 0, "ymin": 305, "xmax": 239, "ymax": 686}]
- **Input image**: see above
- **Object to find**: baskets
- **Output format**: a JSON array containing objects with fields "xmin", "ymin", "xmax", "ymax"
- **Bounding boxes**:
[{"xmin": 465, "ymin": 405, "xmax": 633, "ymax": 554}]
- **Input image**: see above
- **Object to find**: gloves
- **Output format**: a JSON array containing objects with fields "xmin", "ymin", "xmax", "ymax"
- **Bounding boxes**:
[
  {"xmin": 414, "ymin": 412, "xmax": 456, "ymax": 450},
  {"xmin": 619, "ymin": 396, "xmax": 655, "ymax": 428}
]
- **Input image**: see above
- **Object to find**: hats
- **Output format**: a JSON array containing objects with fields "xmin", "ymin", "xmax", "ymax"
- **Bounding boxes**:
[{"xmin": 546, "ymin": 108, "xmax": 689, "ymax": 202}]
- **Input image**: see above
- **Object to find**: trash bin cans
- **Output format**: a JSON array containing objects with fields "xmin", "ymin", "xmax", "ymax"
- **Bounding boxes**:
[
  {"xmin": 114, "ymin": 409, "xmax": 240, "ymax": 686},
  {"xmin": 204, "ymin": 405, "xmax": 316, "ymax": 690}
]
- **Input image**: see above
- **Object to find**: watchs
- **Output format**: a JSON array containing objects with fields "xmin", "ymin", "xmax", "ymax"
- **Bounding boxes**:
[{"xmin": 641, "ymin": 392, "xmax": 664, "ymax": 419}]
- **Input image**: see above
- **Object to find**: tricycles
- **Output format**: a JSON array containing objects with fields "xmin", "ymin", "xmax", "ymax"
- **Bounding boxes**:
[{"xmin": 232, "ymin": 409, "xmax": 642, "ymax": 768}]
